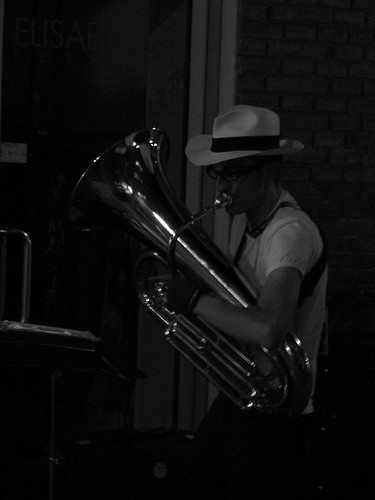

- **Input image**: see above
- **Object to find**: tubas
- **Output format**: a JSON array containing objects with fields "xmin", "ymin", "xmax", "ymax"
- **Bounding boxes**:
[{"xmin": 67, "ymin": 127, "xmax": 312, "ymax": 430}]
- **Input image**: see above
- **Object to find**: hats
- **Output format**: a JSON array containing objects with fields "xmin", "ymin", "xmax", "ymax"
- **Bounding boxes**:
[{"xmin": 184, "ymin": 104, "xmax": 305, "ymax": 167}]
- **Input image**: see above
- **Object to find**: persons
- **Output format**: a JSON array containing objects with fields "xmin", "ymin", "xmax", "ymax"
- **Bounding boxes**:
[{"xmin": 149, "ymin": 105, "xmax": 330, "ymax": 436}]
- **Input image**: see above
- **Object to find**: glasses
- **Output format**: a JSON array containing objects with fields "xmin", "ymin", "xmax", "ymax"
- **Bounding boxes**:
[{"xmin": 206, "ymin": 161, "xmax": 273, "ymax": 182}]
